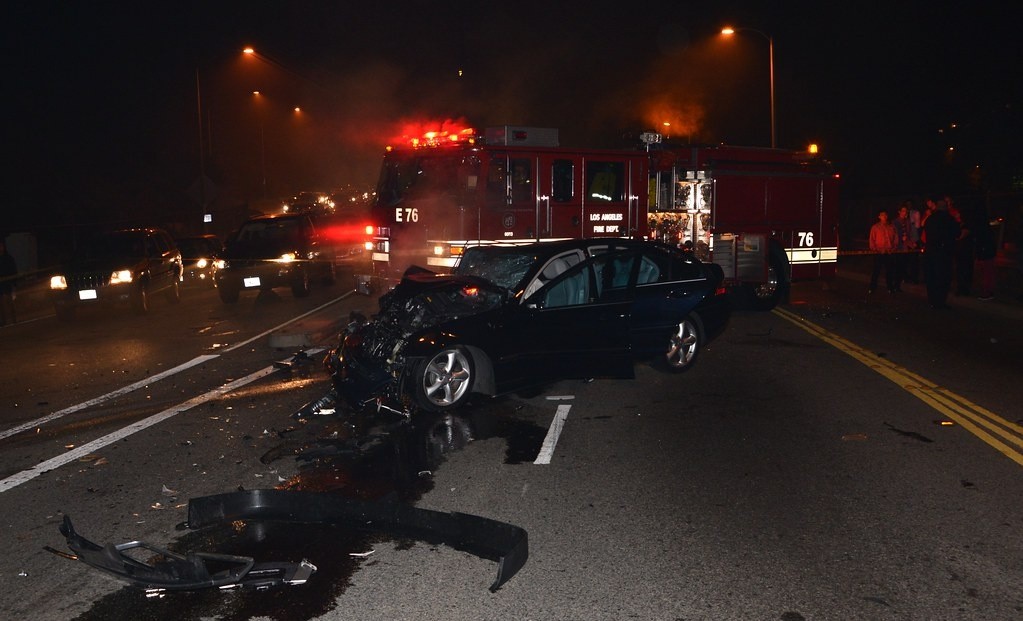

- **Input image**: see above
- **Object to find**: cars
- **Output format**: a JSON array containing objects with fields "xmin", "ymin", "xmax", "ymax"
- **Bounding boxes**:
[
  {"xmin": 285, "ymin": 188, "xmax": 375, "ymax": 231},
  {"xmin": 323, "ymin": 234, "xmax": 730, "ymax": 417},
  {"xmin": 169, "ymin": 232, "xmax": 223, "ymax": 291}
]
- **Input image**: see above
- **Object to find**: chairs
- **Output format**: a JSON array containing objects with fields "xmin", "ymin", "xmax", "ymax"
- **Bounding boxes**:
[{"xmin": 542, "ymin": 258, "xmax": 577, "ymax": 306}]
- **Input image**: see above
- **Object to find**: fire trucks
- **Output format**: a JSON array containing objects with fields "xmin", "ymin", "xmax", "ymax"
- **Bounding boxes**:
[{"xmin": 364, "ymin": 127, "xmax": 841, "ymax": 314}]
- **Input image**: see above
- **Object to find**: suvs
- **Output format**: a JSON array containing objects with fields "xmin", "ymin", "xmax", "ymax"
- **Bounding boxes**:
[
  {"xmin": 50, "ymin": 227, "xmax": 184, "ymax": 320},
  {"xmin": 214, "ymin": 207, "xmax": 336, "ymax": 305}
]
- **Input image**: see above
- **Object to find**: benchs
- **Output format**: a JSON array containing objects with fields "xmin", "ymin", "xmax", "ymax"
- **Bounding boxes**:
[{"xmin": 597, "ymin": 257, "xmax": 656, "ymax": 286}]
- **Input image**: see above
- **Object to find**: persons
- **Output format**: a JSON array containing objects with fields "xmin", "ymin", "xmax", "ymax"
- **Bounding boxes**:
[{"xmin": 869, "ymin": 195, "xmax": 964, "ymax": 307}]
